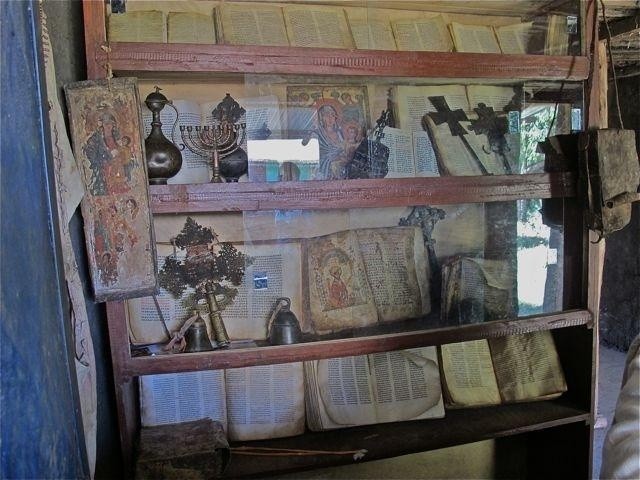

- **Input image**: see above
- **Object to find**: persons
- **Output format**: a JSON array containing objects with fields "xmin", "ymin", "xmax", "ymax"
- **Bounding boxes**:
[{"xmin": 293, "ymin": 91, "xmax": 359, "ymax": 155}]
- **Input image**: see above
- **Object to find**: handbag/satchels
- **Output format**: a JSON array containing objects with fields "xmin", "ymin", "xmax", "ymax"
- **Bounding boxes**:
[{"xmin": 577, "ymin": 126, "xmax": 640, "ymax": 244}]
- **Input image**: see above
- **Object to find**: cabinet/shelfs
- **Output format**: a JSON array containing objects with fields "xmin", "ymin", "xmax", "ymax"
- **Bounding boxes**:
[{"xmin": 83, "ymin": 0, "xmax": 601, "ymax": 480}]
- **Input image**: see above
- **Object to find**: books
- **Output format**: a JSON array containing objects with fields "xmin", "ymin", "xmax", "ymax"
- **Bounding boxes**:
[
  {"xmin": 347, "ymin": 6, "xmax": 455, "ymax": 55},
  {"xmin": 305, "ymin": 346, "xmax": 447, "ymax": 433},
  {"xmin": 438, "ymin": 258, "xmax": 515, "ymax": 324},
  {"xmin": 448, "ymin": 15, "xmax": 545, "ymax": 54},
  {"xmin": 140, "ymin": 92, "xmax": 280, "ymax": 185},
  {"xmin": 215, "ymin": 1, "xmax": 358, "ymax": 48},
  {"xmin": 390, "ymin": 83, "xmax": 521, "ymax": 135},
  {"xmin": 440, "ymin": 327, "xmax": 569, "ymax": 409},
  {"xmin": 285, "ymin": 82, "xmax": 372, "ymax": 179},
  {"xmin": 537, "ymin": 8, "xmax": 576, "ymax": 59},
  {"xmin": 139, "ymin": 361, "xmax": 307, "ymax": 439},
  {"xmin": 423, "ymin": 113, "xmax": 518, "ymax": 177},
  {"xmin": 125, "ymin": 240, "xmax": 304, "ymax": 347},
  {"xmin": 366, "ymin": 124, "xmax": 441, "ymax": 177},
  {"xmin": 107, "ymin": 9, "xmax": 215, "ymax": 42},
  {"xmin": 303, "ymin": 224, "xmax": 432, "ymax": 332}
]
